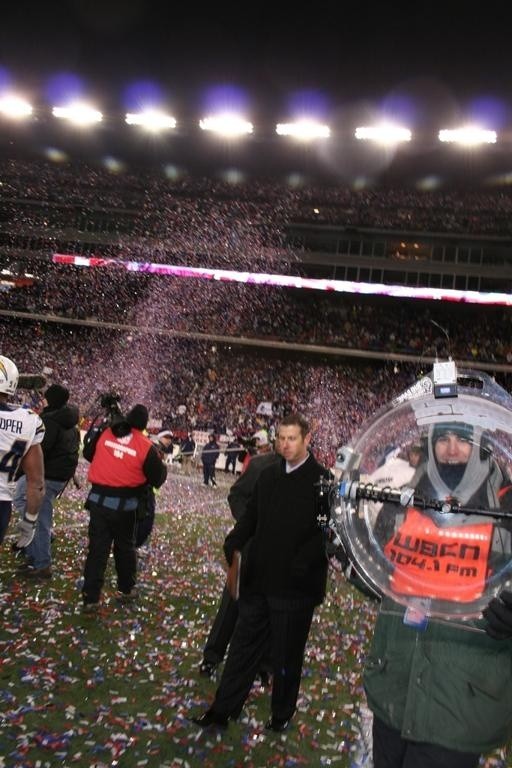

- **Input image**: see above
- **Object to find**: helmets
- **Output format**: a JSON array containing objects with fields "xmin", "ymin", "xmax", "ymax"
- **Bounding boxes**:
[
  {"xmin": 420, "ymin": 421, "xmax": 493, "ymax": 459},
  {"xmin": 384, "ymin": 443, "xmax": 395, "ymax": 455},
  {"xmin": 252, "ymin": 429, "xmax": 268, "ymax": 446},
  {"xmin": 408, "ymin": 442, "xmax": 424, "ymax": 452},
  {"xmin": 0, "ymin": 355, "xmax": 19, "ymax": 396}
]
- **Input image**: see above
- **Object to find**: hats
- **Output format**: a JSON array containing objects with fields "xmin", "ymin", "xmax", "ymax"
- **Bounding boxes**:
[
  {"xmin": 127, "ymin": 404, "xmax": 148, "ymax": 430},
  {"xmin": 45, "ymin": 384, "xmax": 69, "ymax": 409},
  {"xmin": 157, "ymin": 428, "xmax": 173, "ymax": 439}
]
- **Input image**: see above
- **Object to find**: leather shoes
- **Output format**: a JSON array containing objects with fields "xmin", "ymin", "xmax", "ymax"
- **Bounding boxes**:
[
  {"xmin": 200, "ymin": 659, "xmax": 219, "ymax": 677},
  {"xmin": 193, "ymin": 709, "xmax": 229, "ymax": 728},
  {"xmin": 258, "ymin": 671, "xmax": 272, "ymax": 686},
  {"xmin": 266, "ymin": 709, "xmax": 297, "ymax": 731}
]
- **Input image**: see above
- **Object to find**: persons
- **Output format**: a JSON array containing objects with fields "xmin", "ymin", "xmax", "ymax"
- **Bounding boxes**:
[
  {"xmin": 190, "ymin": 411, "xmax": 334, "ymax": 732},
  {"xmin": 14, "ymin": 381, "xmax": 80, "ymax": 580},
  {"xmin": 404, "ymin": 439, "xmax": 422, "ymax": 466},
  {"xmin": 197, "ymin": 448, "xmax": 274, "ymax": 692},
  {"xmin": 1, "ymin": 354, "xmax": 47, "ymax": 557},
  {"xmin": 1, "ymin": 158, "xmax": 512, "ymax": 489},
  {"xmin": 78, "ymin": 401, "xmax": 170, "ymax": 606},
  {"xmin": 362, "ymin": 420, "xmax": 510, "ymax": 766}
]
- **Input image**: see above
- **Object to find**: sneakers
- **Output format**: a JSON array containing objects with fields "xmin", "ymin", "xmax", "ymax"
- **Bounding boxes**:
[{"xmin": 11, "ymin": 528, "xmax": 146, "ymax": 614}]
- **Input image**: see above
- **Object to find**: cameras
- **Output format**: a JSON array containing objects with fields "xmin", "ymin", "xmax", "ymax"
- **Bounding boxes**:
[{"xmin": 238, "ymin": 436, "xmax": 256, "ymax": 452}]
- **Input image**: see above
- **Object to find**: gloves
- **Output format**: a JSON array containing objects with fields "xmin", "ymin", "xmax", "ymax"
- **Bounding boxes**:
[
  {"xmin": 12, "ymin": 515, "xmax": 37, "ymax": 548},
  {"xmin": 482, "ymin": 589, "xmax": 512, "ymax": 640},
  {"xmin": 335, "ymin": 544, "xmax": 395, "ymax": 602}
]
